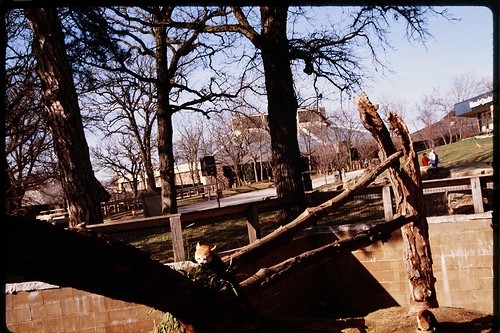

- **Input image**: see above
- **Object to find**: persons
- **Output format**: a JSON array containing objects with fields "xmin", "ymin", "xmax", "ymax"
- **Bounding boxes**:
[
  {"xmin": 428, "ymin": 148, "xmax": 438, "ymax": 167},
  {"xmin": 421, "ymin": 153, "xmax": 429, "ymax": 166}
]
